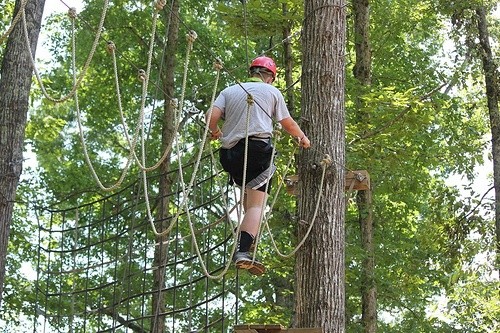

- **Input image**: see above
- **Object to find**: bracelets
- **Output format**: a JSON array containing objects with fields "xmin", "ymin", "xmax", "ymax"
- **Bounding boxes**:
[
  {"xmin": 300, "ymin": 135, "xmax": 305, "ymax": 142},
  {"xmin": 212, "ymin": 128, "xmax": 221, "ymax": 135}
]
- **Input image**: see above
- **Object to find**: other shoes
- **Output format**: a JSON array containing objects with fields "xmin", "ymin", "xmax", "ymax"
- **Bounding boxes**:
[{"xmin": 249, "ymin": 137, "xmax": 270, "ymax": 145}]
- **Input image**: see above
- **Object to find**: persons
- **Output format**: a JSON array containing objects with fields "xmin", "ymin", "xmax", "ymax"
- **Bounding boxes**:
[{"xmin": 206, "ymin": 57, "xmax": 311, "ymax": 276}]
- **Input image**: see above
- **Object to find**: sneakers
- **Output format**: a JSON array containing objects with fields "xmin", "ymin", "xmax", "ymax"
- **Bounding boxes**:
[
  {"xmin": 232, "ymin": 252, "xmax": 266, "ymax": 276},
  {"xmin": 249, "ymin": 243, "xmax": 258, "ymax": 252}
]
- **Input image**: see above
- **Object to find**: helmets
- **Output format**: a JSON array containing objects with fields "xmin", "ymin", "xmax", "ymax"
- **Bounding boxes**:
[{"xmin": 250, "ymin": 56, "xmax": 277, "ymax": 81}]
